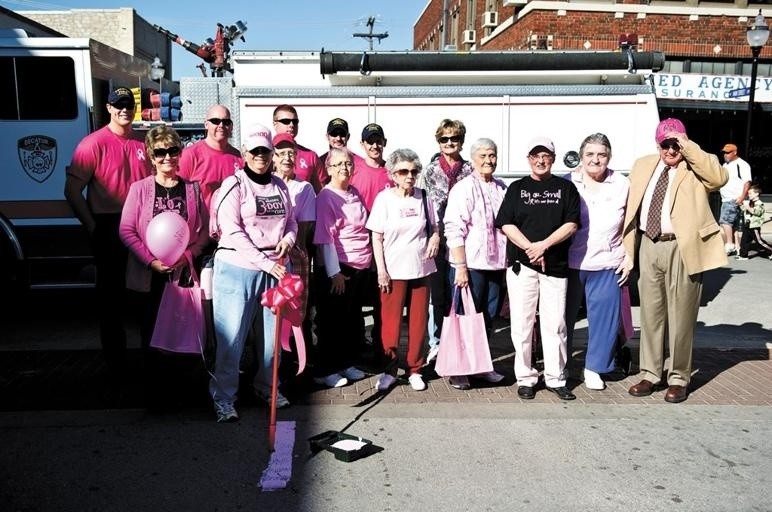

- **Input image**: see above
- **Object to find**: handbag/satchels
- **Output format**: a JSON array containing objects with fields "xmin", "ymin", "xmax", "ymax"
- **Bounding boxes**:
[
  {"xmin": 435, "ymin": 284, "xmax": 494, "ymax": 377},
  {"xmin": 152, "ymin": 250, "xmax": 207, "ymax": 354}
]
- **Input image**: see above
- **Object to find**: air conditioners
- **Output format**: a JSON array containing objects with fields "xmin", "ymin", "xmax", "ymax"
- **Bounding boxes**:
[
  {"xmin": 462, "ymin": 29, "xmax": 475, "ymax": 44},
  {"xmin": 481, "ymin": 12, "xmax": 498, "ymax": 28}
]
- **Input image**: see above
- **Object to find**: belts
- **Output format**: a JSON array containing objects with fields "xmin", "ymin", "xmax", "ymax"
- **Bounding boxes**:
[{"xmin": 640, "ymin": 230, "xmax": 676, "ymax": 242}]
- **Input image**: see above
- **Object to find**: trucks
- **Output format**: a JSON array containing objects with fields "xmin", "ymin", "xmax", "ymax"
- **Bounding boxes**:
[{"xmin": 0, "ymin": 17, "xmax": 666, "ymax": 377}]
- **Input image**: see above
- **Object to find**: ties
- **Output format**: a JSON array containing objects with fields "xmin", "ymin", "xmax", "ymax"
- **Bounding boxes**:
[{"xmin": 646, "ymin": 166, "xmax": 670, "ymax": 242}]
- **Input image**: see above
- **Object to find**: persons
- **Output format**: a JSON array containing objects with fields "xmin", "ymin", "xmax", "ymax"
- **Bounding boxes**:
[
  {"xmin": 715, "ymin": 143, "xmax": 752, "ymax": 256},
  {"xmin": 560, "ymin": 132, "xmax": 634, "ymax": 391},
  {"xmin": 491, "ymin": 137, "xmax": 578, "ymax": 401},
  {"xmin": 65, "ymin": 87, "xmax": 507, "ymax": 423},
  {"xmin": 733, "ymin": 184, "xmax": 772, "ymax": 261},
  {"xmin": 621, "ymin": 116, "xmax": 732, "ymax": 404}
]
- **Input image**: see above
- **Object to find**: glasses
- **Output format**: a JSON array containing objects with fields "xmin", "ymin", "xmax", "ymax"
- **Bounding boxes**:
[
  {"xmin": 438, "ymin": 137, "xmax": 461, "ymax": 142},
  {"xmin": 392, "ymin": 169, "xmax": 419, "ymax": 175},
  {"xmin": 151, "ymin": 146, "xmax": 181, "ymax": 158},
  {"xmin": 111, "ymin": 104, "xmax": 135, "ymax": 109},
  {"xmin": 207, "ymin": 118, "xmax": 232, "ymax": 126},
  {"xmin": 659, "ymin": 142, "xmax": 680, "ymax": 151},
  {"xmin": 276, "ymin": 152, "xmax": 296, "ymax": 157},
  {"xmin": 531, "ymin": 154, "xmax": 552, "ymax": 160},
  {"xmin": 330, "ymin": 162, "xmax": 354, "ymax": 168},
  {"xmin": 275, "ymin": 119, "xmax": 299, "ymax": 125},
  {"xmin": 248, "ymin": 149, "xmax": 270, "ymax": 155}
]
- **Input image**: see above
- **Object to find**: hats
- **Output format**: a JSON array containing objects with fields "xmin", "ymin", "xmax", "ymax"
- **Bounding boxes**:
[
  {"xmin": 655, "ymin": 118, "xmax": 685, "ymax": 143},
  {"xmin": 272, "ymin": 134, "xmax": 295, "ymax": 146},
  {"xmin": 327, "ymin": 118, "xmax": 348, "ymax": 134},
  {"xmin": 527, "ymin": 138, "xmax": 554, "ymax": 157},
  {"xmin": 723, "ymin": 144, "xmax": 736, "ymax": 153},
  {"xmin": 108, "ymin": 87, "xmax": 133, "ymax": 104},
  {"xmin": 244, "ymin": 125, "xmax": 274, "ymax": 152},
  {"xmin": 362, "ymin": 123, "xmax": 384, "ymax": 142}
]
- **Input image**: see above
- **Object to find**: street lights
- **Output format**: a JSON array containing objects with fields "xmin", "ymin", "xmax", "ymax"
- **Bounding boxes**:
[{"xmin": 741, "ymin": 9, "xmax": 771, "ymax": 163}]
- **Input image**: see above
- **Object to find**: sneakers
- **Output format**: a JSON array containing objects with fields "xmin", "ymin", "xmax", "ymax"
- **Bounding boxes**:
[
  {"xmin": 769, "ymin": 254, "xmax": 772, "ymax": 260},
  {"xmin": 484, "ymin": 371, "xmax": 504, "ymax": 382},
  {"xmin": 513, "ymin": 369, "xmax": 690, "ymax": 402},
  {"xmin": 725, "ymin": 244, "xmax": 736, "ymax": 254},
  {"xmin": 449, "ymin": 376, "xmax": 470, "ymax": 389},
  {"xmin": 736, "ymin": 256, "xmax": 748, "ymax": 261},
  {"xmin": 203, "ymin": 365, "xmax": 429, "ymax": 422}
]
